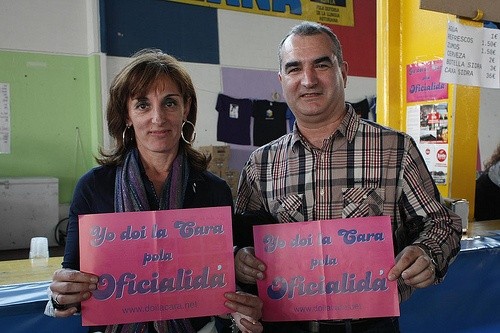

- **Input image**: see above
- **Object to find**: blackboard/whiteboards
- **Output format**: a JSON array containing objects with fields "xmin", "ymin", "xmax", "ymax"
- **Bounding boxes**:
[{"xmin": 0, "ymin": 177, "xmax": 60, "ymax": 251}]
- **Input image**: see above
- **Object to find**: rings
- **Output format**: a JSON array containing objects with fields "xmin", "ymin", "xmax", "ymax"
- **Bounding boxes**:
[{"xmin": 55, "ymin": 293, "xmax": 62, "ymax": 305}]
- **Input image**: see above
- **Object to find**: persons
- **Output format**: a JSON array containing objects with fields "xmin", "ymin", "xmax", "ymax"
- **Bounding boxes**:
[
  {"xmin": 50, "ymin": 48, "xmax": 264, "ymax": 333},
  {"xmin": 233, "ymin": 21, "xmax": 462, "ymax": 333}
]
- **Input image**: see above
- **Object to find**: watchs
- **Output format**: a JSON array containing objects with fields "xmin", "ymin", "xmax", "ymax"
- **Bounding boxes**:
[{"xmin": 414, "ymin": 243, "xmax": 438, "ymax": 270}]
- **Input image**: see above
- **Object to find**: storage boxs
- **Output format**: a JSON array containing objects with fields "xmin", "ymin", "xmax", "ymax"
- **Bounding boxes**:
[
  {"xmin": 0, "ymin": 176, "xmax": 60, "ymax": 250},
  {"xmin": 197, "ymin": 145, "xmax": 238, "ymax": 206}
]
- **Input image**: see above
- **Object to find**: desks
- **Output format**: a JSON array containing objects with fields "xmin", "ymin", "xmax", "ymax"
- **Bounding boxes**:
[{"xmin": 0, "ymin": 219, "xmax": 500, "ymax": 333}]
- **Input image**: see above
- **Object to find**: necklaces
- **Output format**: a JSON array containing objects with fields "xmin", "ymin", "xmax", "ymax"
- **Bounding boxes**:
[{"xmin": 149, "ymin": 182, "xmax": 159, "ymax": 203}]
